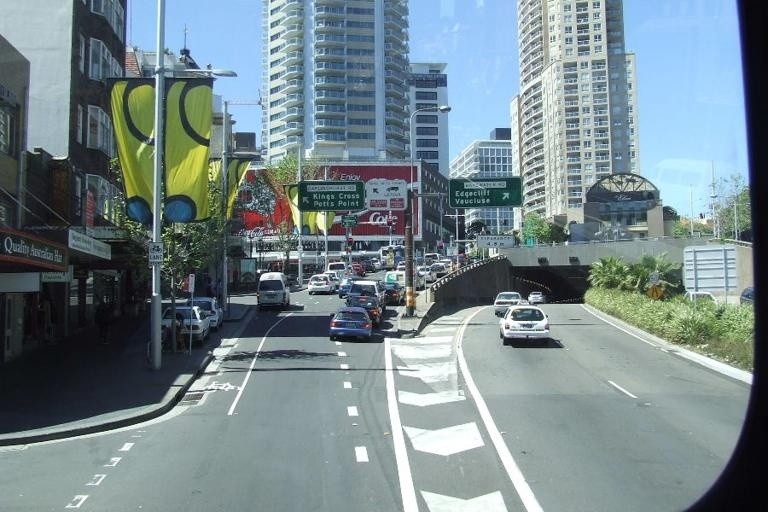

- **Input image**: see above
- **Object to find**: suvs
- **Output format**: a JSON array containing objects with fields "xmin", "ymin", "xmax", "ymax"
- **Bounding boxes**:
[
  {"xmin": 739, "ymin": 286, "xmax": 754, "ymax": 306},
  {"xmin": 322, "ymin": 270, "xmax": 342, "ymax": 288}
]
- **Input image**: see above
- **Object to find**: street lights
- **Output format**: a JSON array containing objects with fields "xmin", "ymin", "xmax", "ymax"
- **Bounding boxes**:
[
  {"xmin": 408, "ymin": 104, "xmax": 453, "ymax": 241},
  {"xmin": 709, "ymin": 193, "xmax": 739, "ymax": 241},
  {"xmin": 218, "ymin": 144, "xmax": 266, "ymax": 313},
  {"xmin": 148, "ymin": 62, "xmax": 241, "ymax": 367}
]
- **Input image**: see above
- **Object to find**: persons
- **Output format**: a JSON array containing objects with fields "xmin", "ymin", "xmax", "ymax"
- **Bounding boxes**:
[
  {"xmin": 233, "ymin": 268, "xmax": 239, "ymax": 290},
  {"xmin": 176, "ymin": 311, "xmax": 185, "ymax": 353},
  {"xmin": 161, "ymin": 324, "xmax": 173, "ymax": 353}
]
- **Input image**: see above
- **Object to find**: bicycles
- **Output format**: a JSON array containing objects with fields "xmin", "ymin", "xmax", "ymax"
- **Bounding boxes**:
[{"xmin": 145, "ymin": 325, "xmax": 169, "ymax": 365}]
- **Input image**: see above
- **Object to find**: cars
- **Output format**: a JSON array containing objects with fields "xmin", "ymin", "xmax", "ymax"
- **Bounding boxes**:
[
  {"xmin": 185, "ymin": 297, "xmax": 223, "ymax": 330},
  {"xmin": 338, "ymin": 276, "xmax": 361, "ymax": 298},
  {"xmin": 495, "ymin": 305, "xmax": 553, "ymax": 344},
  {"xmin": 527, "ymin": 291, "xmax": 548, "ymax": 305},
  {"xmin": 682, "ymin": 291, "xmax": 717, "ymax": 309},
  {"xmin": 344, "ymin": 296, "xmax": 383, "ymax": 327},
  {"xmin": 307, "ymin": 274, "xmax": 336, "ymax": 295},
  {"xmin": 348, "ymin": 245, "xmax": 490, "ymax": 292},
  {"xmin": 161, "ymin": 306, "xmax": 211, "ymax": 346},
  {"xmin": 328, "ymin": 307, "xmax": 374, "ymax": 341},
  {"xmin": 490, "ymin": 290, "xmax": 522, "ymax": 315},
  {"xmin": 384, "ymin": 282, "xmax": 405, "ymax": 306}
]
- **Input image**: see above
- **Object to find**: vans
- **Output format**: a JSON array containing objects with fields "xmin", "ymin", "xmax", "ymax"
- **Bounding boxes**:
[
  {"xmin": 345, "ymin": 280, "xmax": 387, "ymax": 312},
  {"xmin": 256, "ymin": 272, "xmax": 292, "ymax": 308},
  {"xmin": 326, "ymin": 261, "xmax": 347, "ymax": 275}
]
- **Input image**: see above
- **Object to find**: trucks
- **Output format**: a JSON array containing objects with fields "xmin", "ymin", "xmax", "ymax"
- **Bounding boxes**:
[{"xmin": 267, "ymin": 259, "xmax": 298, "ymax": 275}]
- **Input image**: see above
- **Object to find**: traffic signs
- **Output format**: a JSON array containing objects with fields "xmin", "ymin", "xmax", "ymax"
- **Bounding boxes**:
[
  {"xmin": 340, "ymin": 214, "xmax": 358, "ymax": 228},
  {"xmin": 448, "ymin": 176, "xmax": 525, "ymax": 209},
  {"xmin": 297, "ymin": 180, "xmax": 365, "ymax": 213}
]
